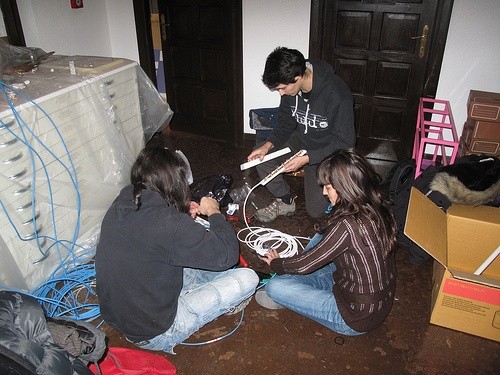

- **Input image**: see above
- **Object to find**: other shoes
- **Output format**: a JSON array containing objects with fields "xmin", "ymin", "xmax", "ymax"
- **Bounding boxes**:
[
  {"xmin": 254, "ymin": 194, "xmax": 297, "ymax": 222},
  {"xmin": 225, "ymin": 295, "xmax": 253, "ymax": 315},
  {"xmin": 255, "ymin": 288, "xmax": 285, "ymax": 309}
]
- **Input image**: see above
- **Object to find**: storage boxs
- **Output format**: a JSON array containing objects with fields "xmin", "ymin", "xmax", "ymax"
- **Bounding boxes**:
[{"xmin": 404, "ymin": 89, "xmax": 500, "ymax": 342}]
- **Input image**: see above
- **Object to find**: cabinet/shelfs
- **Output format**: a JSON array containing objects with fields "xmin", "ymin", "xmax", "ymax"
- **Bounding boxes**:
[
  {"xmin": 0, "ymin": 55, "xmax": 145, "ymax": 291},
  {"xmin": 412, "ymin": 98, "xmax": 459, "ymax": 179}
]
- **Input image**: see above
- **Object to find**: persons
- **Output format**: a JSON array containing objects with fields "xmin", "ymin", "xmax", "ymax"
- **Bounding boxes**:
[
  {"xmin": 97, "ymin": 144, "xmax": 259, "ymax": 354},
  {"xmin": 247, "ymin": 47, "xmax": 355, "ymax": 222},
  {"xmin": 256, "ymin": 150, "xmax": 396, "ymax": 336}
]
